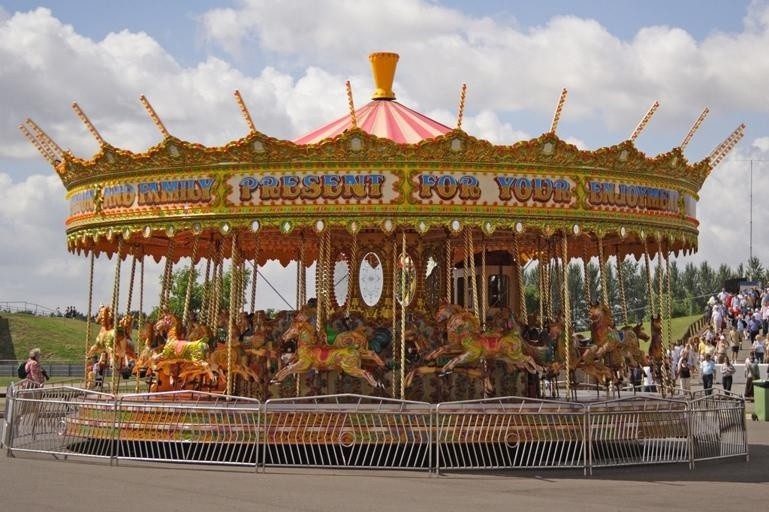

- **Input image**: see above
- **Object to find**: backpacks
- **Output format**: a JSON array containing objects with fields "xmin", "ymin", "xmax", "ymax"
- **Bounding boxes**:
[{"xmin": 17, "ymin": 360, "xmax": 30, "ymax": 379}]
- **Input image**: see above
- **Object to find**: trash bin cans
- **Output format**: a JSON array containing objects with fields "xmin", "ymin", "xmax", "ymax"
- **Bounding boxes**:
[{"xmin": 751, "ymin": 379, "xmax": 769, "ymax": 422}]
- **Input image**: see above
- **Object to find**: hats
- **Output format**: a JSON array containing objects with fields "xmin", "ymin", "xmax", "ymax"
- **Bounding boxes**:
[{"xmin": 719, "ymin": 335, "xmax": 725, "ymax": 340}]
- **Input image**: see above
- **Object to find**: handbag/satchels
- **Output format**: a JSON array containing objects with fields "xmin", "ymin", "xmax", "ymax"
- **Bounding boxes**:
[
  {"xmin": 752, "ymin": 375, "xmax": 759, "ymax": 379},
  {"xmin": 720, "ymin": 321, "xmax": 726, "ymax": 328},
  {"xmin": 752, "ymin": 346, "xmax": 756, "ymax": 351},
  {"xmin": 727, "ymin": 367, "xmax": 734, "ymax": 373}
]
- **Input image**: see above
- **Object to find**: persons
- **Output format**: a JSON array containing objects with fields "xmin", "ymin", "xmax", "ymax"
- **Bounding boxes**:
[
  {"xmin": 19, "ymin": 348, "xmax": 44, "ymax": 425},
  {"xmin": 123, "ymin": 363, "xmax": 132, "ymax": 379},
  {"xmin": 92, "ymin": 356, "xmax": 102, "ymax": 387},
  {"xmin": 633, "ymin": 288, "xmax": 769, "ymax": 401}
]
